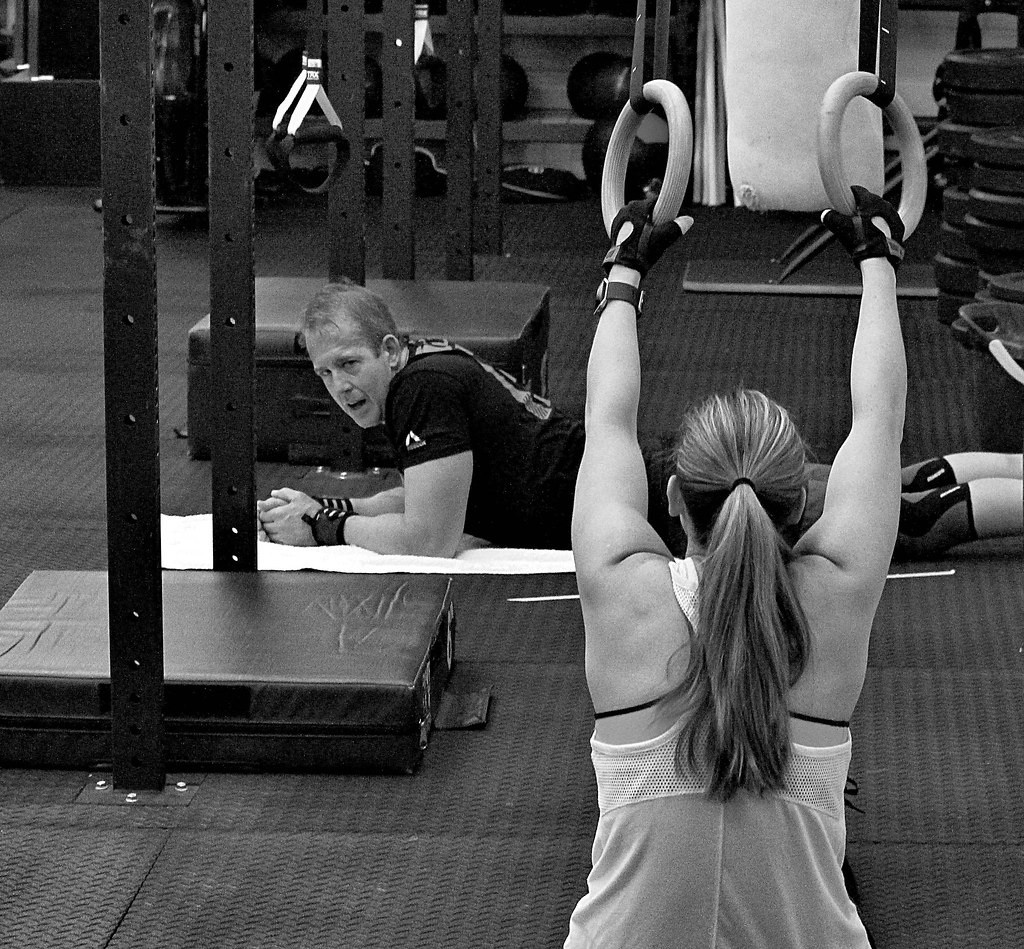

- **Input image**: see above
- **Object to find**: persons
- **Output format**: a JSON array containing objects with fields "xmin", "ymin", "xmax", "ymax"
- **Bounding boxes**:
[
  {"xmin": 257, "ymin": 282, "xmax": 1024, "ymax": 560},
  {"xmin": 556, "ymin": 185, "xmax": 907, "ymax": 949}
]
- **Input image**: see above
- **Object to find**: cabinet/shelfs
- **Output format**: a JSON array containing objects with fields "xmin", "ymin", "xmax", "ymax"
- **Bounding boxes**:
[{"xmin": 276, "ymin": 10, "xmax": 677, "ymax": 142}]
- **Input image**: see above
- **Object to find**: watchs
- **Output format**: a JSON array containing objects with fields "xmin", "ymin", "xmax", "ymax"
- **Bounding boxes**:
[{"xmin": 592, "ymin": 278, "xmax": 646, "ymax": 319}]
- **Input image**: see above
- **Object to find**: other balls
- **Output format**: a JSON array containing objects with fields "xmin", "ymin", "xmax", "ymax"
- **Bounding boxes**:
[{"xmin": 199, "ymin": 52, "xmax": 672, "ymax": 202}]
